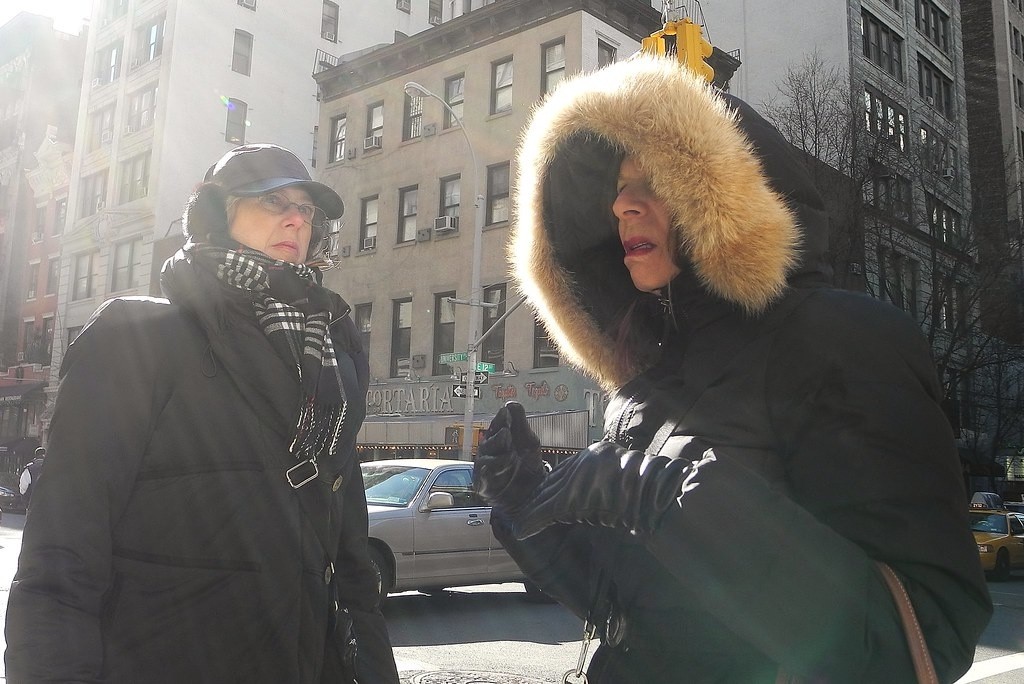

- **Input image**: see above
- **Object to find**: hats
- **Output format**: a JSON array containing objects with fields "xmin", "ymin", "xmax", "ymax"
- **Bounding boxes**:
[{"xmin": 210, "ymin": 144, "xmax": 344, "ymax": 220}]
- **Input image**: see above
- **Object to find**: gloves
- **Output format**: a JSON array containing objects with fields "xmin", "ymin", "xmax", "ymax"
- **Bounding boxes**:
[
  {"xmin": 472, "ymin": 401, "xmax": 575, "ymax": 576},
  {"xmin": 510, "ymin": 441, "xmax": 694, "ymax": 541}
]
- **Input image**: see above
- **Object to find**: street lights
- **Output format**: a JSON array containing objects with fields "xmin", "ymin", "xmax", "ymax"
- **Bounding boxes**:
[{"xmin": 404, "ymin": 83, "xmax": 484, "ymax": 463}]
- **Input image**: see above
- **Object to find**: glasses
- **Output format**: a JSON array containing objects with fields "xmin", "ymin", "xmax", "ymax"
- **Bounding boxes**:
[{"xmin": 258, "ymin": 192, "xmax": 328, "ymax": 227}]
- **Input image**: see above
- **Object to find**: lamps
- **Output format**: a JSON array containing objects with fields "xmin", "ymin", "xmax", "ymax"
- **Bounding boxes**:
[{"xmin": 503, "ymin": 361, "xmax": 519, "ymax": 375}]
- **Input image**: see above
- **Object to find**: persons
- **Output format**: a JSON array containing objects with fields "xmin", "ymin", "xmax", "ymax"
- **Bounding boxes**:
[
  {"xmin": 470, "ymin": 49, "xmax": 993, "ymax": 684},
  {"xmin": 3, "ymin": 144, "xmax": 401, "ymax": 684},
  {"xmin": 18, "ymin": 447, "xmax": 46, "ymax": 509}
]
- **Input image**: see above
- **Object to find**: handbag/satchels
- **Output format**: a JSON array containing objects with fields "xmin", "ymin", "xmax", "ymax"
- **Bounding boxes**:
[{"xmin": 332, "ymin": 606, "xmax": 360, "ymax": 684}]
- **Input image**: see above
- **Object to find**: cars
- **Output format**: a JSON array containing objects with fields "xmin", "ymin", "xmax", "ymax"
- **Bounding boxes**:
[
  {"xmin": 0, "ymin": 485, "xmax": 23, "ymax": 512},
  {"xmin": 360, "ymin": 459, "xmax": 560, "ymax": 609},
  {"xmin": 968, "ymin": 492, "xmax": 1024, "ymax": 581}
]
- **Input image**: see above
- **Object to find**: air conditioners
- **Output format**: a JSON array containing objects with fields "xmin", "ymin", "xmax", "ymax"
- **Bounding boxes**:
[
  {"xmin": 31, "ymin": 232, "xmax": 41, "ymax": 242},
  {"xmin": 97, "ymin": 200, "xmax": 105, "ymax": 210},
  {"xmin": 28, "ymin": 290, "xmax": 36, "ymax": 299},
  {"xmin": 398, "ymin": 0, "xmax": 410, "ymax": 12},
  {"xmin": 433, "ymin": 216, "xmax": 455, "ymax": 232},
  {"xmin": 240, "ymin": 0, "xmax": 255, "ymax": 9},
  {"xmin": 324, "ymin": 31, "xmax": 335, "ymax": 41},
  {"xmin": 363, "ymin": 237, "xmax": 376, "ymax": 249},
  {"xmin": 123, "ymin": 125, "xmax": 135, "ymax": 136},
  {"xmin": 91, "ymin": 77, "xmax": 102, "ymax": 89},
  {"xmin": 102, "ymin": 131, "xmax": 112, "ymax": 143},
  {"xmin": 100, "ymin": 19, "xmax": 109, "ymax": 28},
  {"xmin": 131, "ymin": 59, "xmax": 140, "ymax": 69},
  {"xmin": 363, "ymin": 135, "xmax": 381, "ymax": 150},
  {"xmin": 942, "ymin": 167, "xmax": 955, "ymax": 180},
  {"xmin": 924, "ymin": 96, "xmax": 934, "ymax": 106},
  {"xmin": 430, "ymin": 15, "xmax": 441, "ymax": 25}
]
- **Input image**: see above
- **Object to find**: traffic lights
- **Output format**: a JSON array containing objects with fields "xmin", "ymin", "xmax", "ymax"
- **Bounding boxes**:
[{"xmin": 675, "ymin": 17, "xmax": 715, "ymax": 83}]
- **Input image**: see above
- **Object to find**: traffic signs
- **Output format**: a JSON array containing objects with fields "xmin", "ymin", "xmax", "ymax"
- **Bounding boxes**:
[
  {"xmin": 452, "ymin": 385, "xmax": 480, "ymax": 399},
  {"xmin": 461, "ymin": 371, "xmax": 489, "ymax": 384}
]
